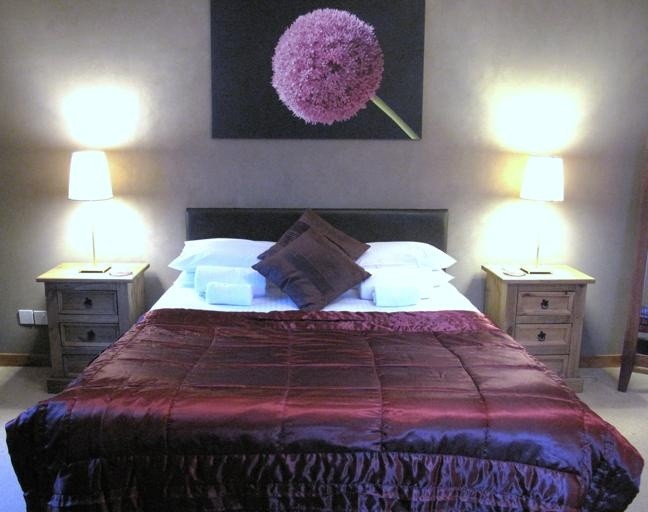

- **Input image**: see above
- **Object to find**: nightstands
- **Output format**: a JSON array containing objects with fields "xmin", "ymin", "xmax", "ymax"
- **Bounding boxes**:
[
  {"xmin": 481, "ymin": 261, "xmax": 594, "ymax": 393},
  {"xmin": 32, "ymin": 258, "xmax": 151, "ymax": 394}
]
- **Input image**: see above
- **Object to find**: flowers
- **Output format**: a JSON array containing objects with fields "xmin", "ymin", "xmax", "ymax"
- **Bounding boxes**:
[{"xmin": 271, "ymin": 9, "xmax": 420, "ymax": 140}]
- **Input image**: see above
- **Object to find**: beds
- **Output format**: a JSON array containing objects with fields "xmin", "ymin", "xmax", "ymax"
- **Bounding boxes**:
[{"xmin": 4, "ymin": 207, "xmax": 647, "ymax": 512}]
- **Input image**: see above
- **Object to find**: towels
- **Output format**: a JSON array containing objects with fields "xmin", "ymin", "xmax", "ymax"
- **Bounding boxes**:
[
  {"xmin": 367, "ymin": 285, "xmax": 422, "ymax": 307},
  {"xmin": 205, "ymin": 282, "xmax": 251, "ymax": 306},
  {"xmin": 357, "ymin": 272, "xmax": 434, "ymax": 296},
  {"xmin": 194, "ymin": 265, "xmax": 241, "ymax": 291}
]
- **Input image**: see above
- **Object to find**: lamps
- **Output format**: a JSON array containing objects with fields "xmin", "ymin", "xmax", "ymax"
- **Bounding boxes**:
[
  {"xmin": 517, "ymin": 157, "xmax": 565, "ymax": 272},
  {"xmin": 67, "ymin": 150, "xmax": 115, "ymax": 275}
]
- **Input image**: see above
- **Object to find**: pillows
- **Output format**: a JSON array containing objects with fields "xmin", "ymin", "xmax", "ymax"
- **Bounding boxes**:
[
  {"xmin": 356, "ymin": 242, "xmax": 456, "ymax": 269},
  {"xmin": 166, "ymin": 237, "xmax": 277, "ymax": 273},
  {"xmin": 363, "ymin": 268, "xmax": 458, "ymax": 289},
  {"xmin": 256, "ymin": 209, "xmax": 370, "ymax": 275},
  {"xmin": 251, "ymin": 233, "xmax": 370, "ymax": 313}
]
